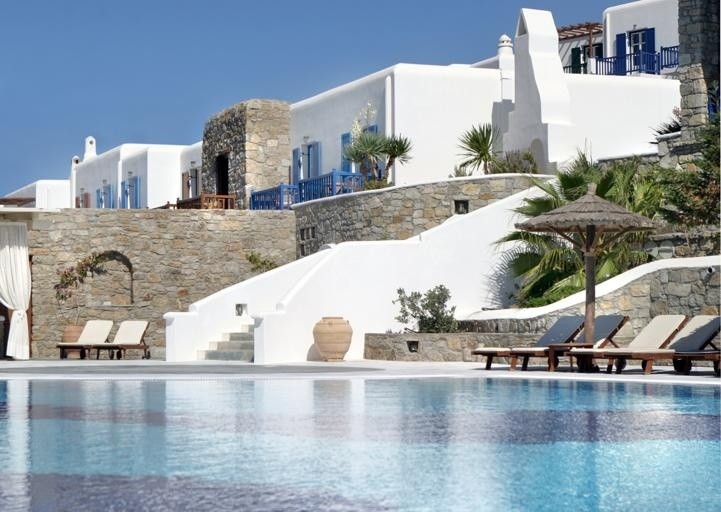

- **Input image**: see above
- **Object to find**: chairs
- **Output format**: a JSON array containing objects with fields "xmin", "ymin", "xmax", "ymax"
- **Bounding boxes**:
[
  {"xmin": 497, "ymin": 315, "xmax": 629, "ymax": 372},
  {"xmin": 564, "ymin": 315, "xmax": 686, "ymax": 374},
  {"xmin": 82, "ymin": 321, "xmax": 151, "ymax": 360},
  {"xmin": 56, "ymin": 319, "xmax": 113, "ymax": 359},
  {"xmin": 603, "ymin": 315, "xmax": 721, "ymax": 372},
  {"xmin": 471, "ymin": 314, "xmax": 585, "ymax": 370}
]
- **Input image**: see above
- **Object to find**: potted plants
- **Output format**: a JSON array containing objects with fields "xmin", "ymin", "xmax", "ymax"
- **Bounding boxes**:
[{"xmin": 54, "ymin": 251, "xmax": 111, "ymax": 359}]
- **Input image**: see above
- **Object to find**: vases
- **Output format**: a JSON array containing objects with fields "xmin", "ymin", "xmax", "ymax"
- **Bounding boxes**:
[{"xmin": 313, "ymin": 316, "xmax": 354, "ymax": 362}]
